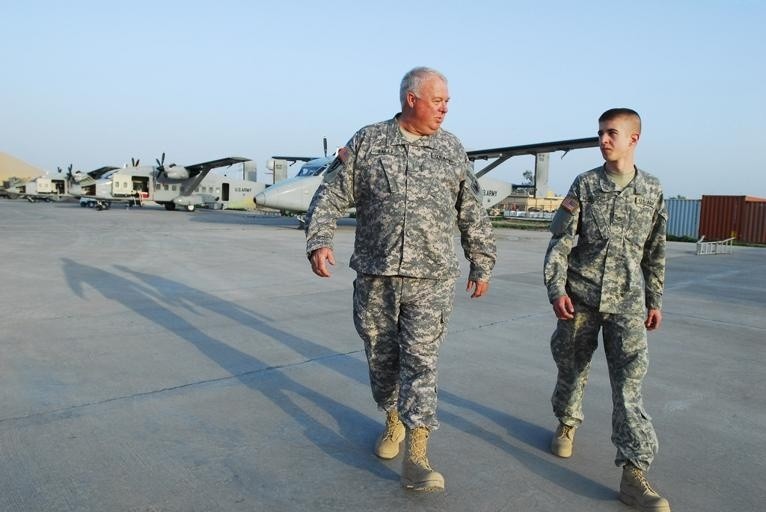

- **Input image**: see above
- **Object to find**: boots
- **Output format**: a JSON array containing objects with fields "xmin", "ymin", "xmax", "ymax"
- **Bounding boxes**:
[
  {"xmin": 402, "ymin": 428, "xmax": 445, "ymax": 493},
  {"xmin": 373, "ymin": 408, "xmax": 406, "ymax": 459},
  {"xmin": 617, "ymin": 466, "xmax": 671, "ymax": 512}
]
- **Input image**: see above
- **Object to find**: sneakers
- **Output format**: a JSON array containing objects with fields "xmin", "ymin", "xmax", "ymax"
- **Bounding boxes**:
[{"xmin": 550, "ymin": 424, "xmax": 575, "ymax": 458}]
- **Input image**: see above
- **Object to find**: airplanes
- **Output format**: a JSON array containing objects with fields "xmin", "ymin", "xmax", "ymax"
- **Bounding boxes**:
[
  {"xmin": 250, "ymin": 136, "xmax": 601, "ymax": 228},
  {"xmin": 0, "ymin": 156, "xmax": 266, "ymax": 212}
]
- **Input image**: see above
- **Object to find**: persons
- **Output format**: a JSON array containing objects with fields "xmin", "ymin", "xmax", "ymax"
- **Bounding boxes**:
[
  {"xmin": 543, "ymin": 105, "xmax": 670, "ymax": 512},
  {"xmin": 304, "ymin": 65, "xmax": 498, "ymax": 495}
]
